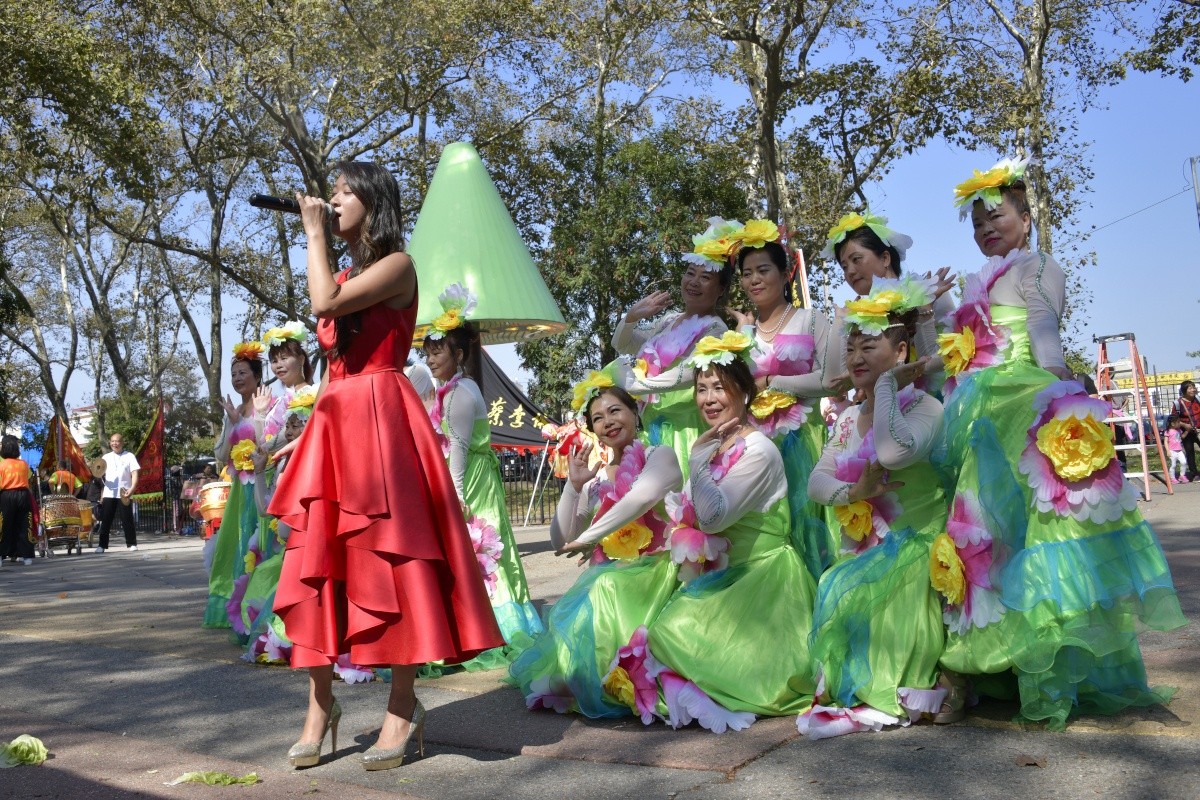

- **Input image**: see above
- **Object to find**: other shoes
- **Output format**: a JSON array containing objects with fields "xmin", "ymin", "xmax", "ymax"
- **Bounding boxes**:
[
  {"xmin": 1177, "ymin": 475, "xmax": 1190, "ymax": 483},
  {"xmin": 95, "ymin": 547, "xmax": 104, "ymax": 553},
  {"xmin": 24, "ymin": 559, "xmax": 32, "ymax": 565},
  {"xmin": 933, "ymin": 684, "xmax": 968, "ymax": 723},
  {"xmin": 1170, "ymin": 478, "xmax": 1180, "ymax": 484},
  {"xmin": 1192, "ymin": 476, "xmax": 1200, "ymax": 482},
  {"xmin": 130, "ymin": 545, "xmax": 137, "ymax": 550}
]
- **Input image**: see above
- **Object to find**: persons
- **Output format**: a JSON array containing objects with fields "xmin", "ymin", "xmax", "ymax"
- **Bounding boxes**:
[
  {"xmin": 0, "ymin": 429, "xmax": 141, "ymax": 567},
  {"xmin": 169, "ymin": 177, "xmax": 1200, "ymax": 741},
  {"xmin": 285, "ymin": 162, "xmax": 425, "ymax": 773}
]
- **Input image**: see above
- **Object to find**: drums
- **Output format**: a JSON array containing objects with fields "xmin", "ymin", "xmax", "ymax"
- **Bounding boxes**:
[
  {"xmin": 36, "ymin": 495, "xmax": 82, "ymax": 539},
  {"xmin": 77, "ymin": 500, "xmax": 92, "ymax": 541},
  {"xmin": 199, "ymin": 482, "xmax": 232, "ymax": 522}
]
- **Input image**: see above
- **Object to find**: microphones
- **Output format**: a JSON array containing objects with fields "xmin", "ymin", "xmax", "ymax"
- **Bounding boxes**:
[{"xmin": 249, "ymin": 193, "xmax": 336, "ymax": 223}]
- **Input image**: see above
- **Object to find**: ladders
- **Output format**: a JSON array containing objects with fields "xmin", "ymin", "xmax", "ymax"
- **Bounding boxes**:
[{"xmin": 1095, "ymin": 333, "xmax": 1173, "ymax": 502}]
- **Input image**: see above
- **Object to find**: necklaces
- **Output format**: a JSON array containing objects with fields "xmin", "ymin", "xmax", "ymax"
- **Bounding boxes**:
[
  {"xmin": 755, "ymin": 302, "xmax": 791, "ymax": 332},
  {"xmin": 754, "ymin": 303, "xmax": 794, "ymax": 341}
]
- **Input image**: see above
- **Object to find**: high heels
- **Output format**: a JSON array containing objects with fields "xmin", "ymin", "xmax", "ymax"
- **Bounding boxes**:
[
  {"xmin": 361, "ymin": 699, "xmax": 426, "ymax": 771},
  {"xmin": 288, "ymin": 695, "xmax": 342, "ymax": 767}
]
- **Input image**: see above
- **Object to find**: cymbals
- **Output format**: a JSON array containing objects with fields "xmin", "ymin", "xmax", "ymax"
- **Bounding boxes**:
[
  {"xmin": 90, "ymin": 458, "xmax": 108, "ymax": 478},
  {"xmin": 120, "ymin": 486, "xmax": 130, "ymax": 506}
]
